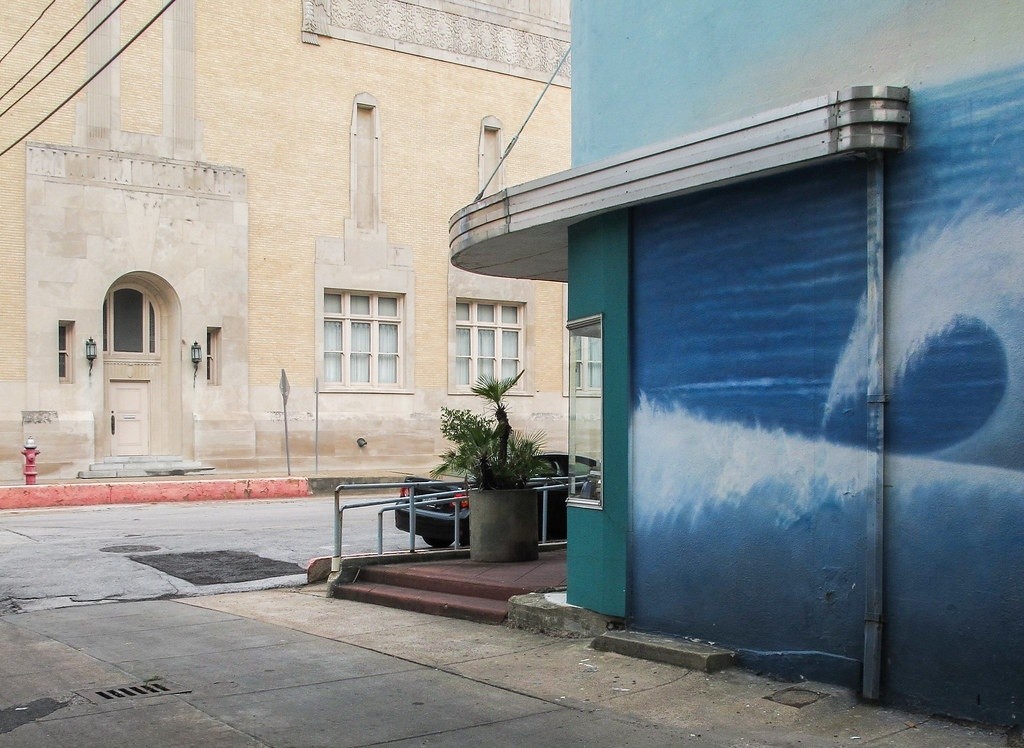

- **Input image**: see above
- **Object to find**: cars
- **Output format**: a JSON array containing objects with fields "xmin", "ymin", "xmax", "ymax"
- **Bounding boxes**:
[{"xmin": 392, "ymin": 449, "xmax": 605, "ymax": 547}]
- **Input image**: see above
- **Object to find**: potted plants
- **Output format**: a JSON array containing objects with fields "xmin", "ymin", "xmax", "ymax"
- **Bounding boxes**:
[{"xmin": 427, "ymin": 367, "xmax": 559, "ymax": 563}]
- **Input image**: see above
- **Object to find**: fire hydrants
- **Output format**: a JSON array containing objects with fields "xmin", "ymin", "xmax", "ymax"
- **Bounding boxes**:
[{"xmin": 21, "ymin": 435, "xmax": 40, "ymax": 485}]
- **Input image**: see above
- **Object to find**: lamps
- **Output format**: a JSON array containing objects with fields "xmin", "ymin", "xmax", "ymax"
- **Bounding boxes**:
[
  {"xmin": 191, "ymin": 341, "xmax": 203, "ymax": 380},
  {"xmin": 86, "ymin": 336, "xmax": 98, "ymax": 377}
]
